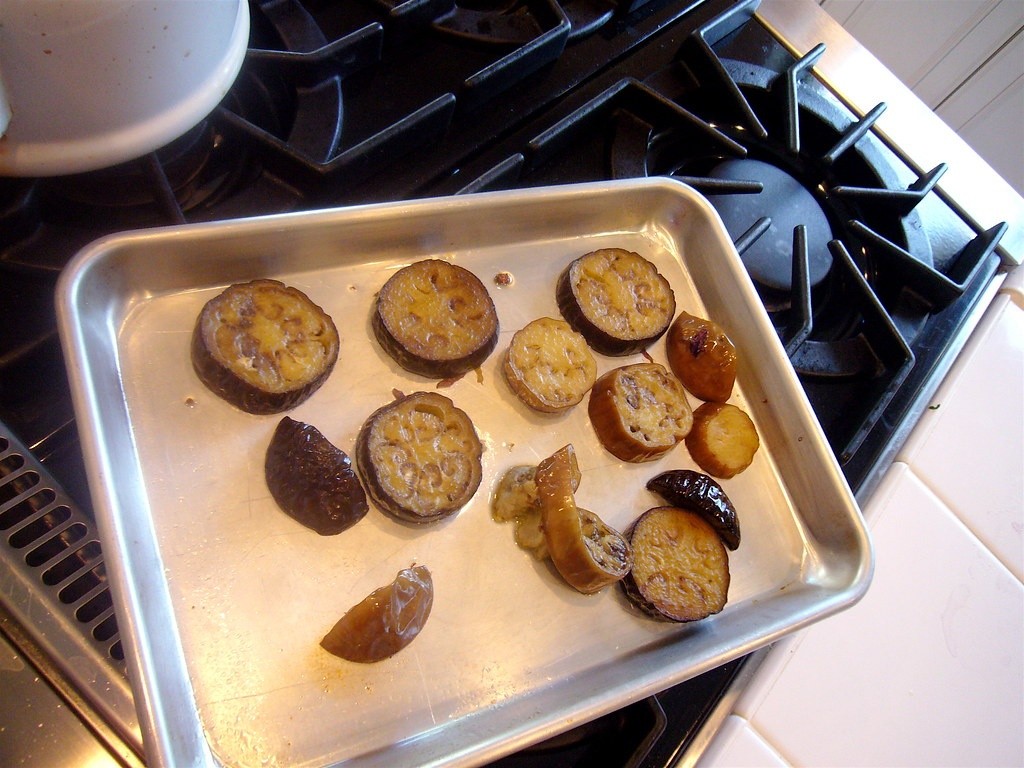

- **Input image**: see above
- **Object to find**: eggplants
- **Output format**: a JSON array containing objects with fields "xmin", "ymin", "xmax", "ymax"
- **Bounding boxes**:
[{"xmin": 189, "ymin": 247, "xmax": 759, "ymax": 663}]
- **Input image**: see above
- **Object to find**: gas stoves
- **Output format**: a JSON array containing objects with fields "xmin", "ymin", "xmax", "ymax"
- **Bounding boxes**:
[{"xmin": 0, "ymin": 2, "xmax": 1021, "ymax": 768}]
